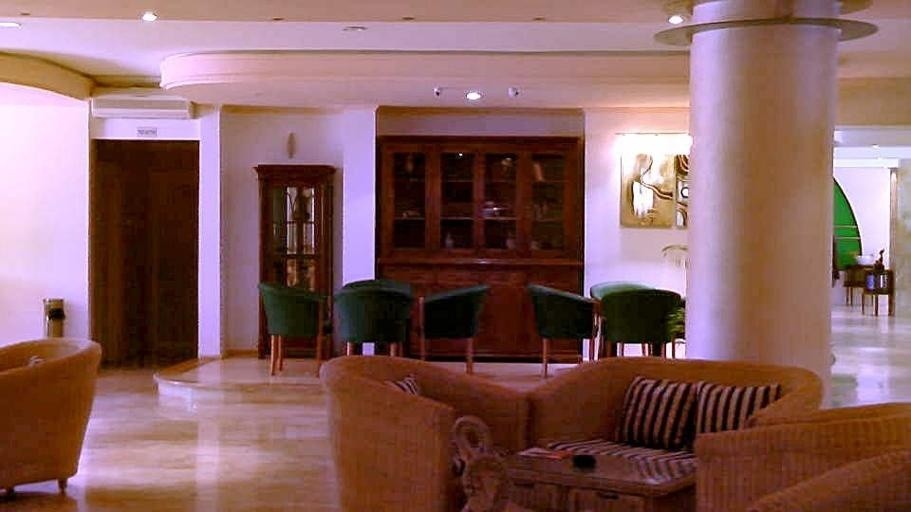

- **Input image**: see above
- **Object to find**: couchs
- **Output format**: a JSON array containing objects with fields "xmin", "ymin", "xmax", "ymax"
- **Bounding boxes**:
[
  {"xmin": 319, "ymin": 356, "xmax": 530, "ymax": 511},
  {"xmin": 530, "ymin": 356, "xmax": 824, "ymax": 498},
  {"xmin": 693, "ymin": 402, "xmax": 910, "ymax": 512}
]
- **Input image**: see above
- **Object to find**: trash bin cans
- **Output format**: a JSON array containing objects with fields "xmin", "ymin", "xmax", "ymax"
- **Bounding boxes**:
[{"xmin": 43, "ymin": 298, "xmax": 64, "ymax": 337}]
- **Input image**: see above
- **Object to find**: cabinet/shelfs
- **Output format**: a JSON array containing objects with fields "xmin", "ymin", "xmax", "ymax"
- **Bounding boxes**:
[
  {"xmin": 373, "ymin": 134, "xmax": 584, "ymax": 363},
  {"xmin": 252, "ymin": 163, "xmax": 332, "ymax": 359}
]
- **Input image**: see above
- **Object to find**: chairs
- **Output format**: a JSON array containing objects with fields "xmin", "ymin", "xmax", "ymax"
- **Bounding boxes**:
[
  {"xmin": 256, "ymin": 277, "xmax": 489, "ymax": 379},
  {"xmin": 528, "ymin": 280, "xmax": 685, "ymax": 377},
  {"xmin": 0, "ymin": 336, "xmax": 105, "ymax": 498}
]
